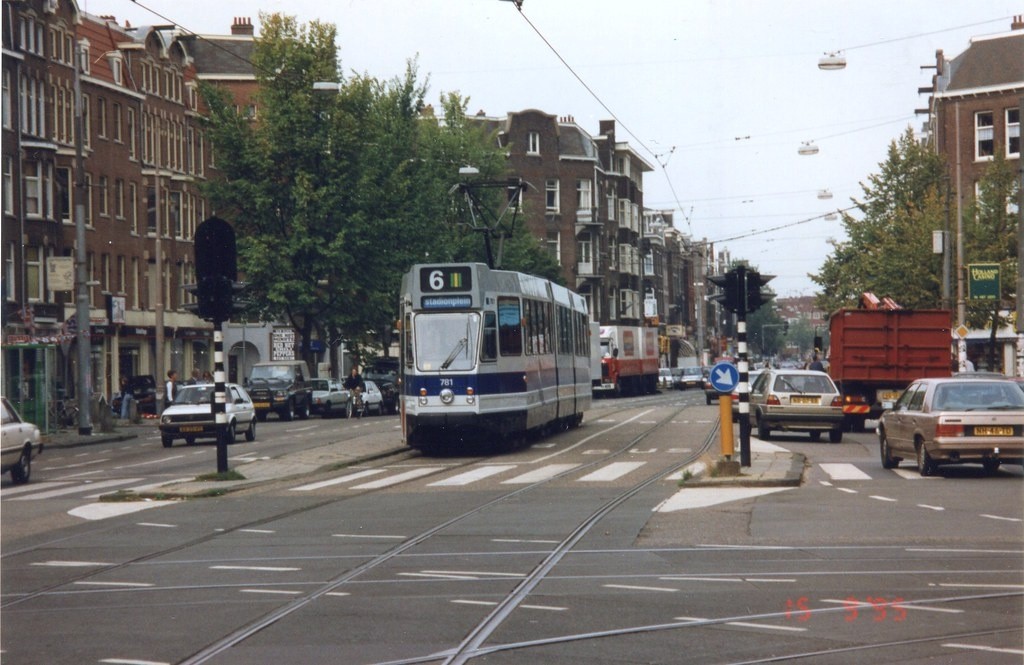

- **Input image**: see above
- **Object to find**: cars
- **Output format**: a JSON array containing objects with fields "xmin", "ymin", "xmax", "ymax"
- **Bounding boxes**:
[
  {"xmin": 0, "ymin": 396, "xmax": 42, "ymax": 484},
  {"xmin": 731, "ymin": 359, "xmax": 845, "ymax": 444},
  {"xmin": 658, "ymin": 365, "xmax": 714, "ymax": 392},
  {"xmin": 307, "ymin": 373, "xmax": 400, "ymax": 419},
  {"xmin": 876, "ymin": 371, "xmax": 1024, "ymax": 476},
  {"xmin": 159, "ymin": 380, "xmax": 257, "ymax": 447}
]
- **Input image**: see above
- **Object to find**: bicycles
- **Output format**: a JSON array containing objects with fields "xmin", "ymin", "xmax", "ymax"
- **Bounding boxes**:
[{"xmin": 344, "ymin": 388, "xmax": 365, "ymax": 419}]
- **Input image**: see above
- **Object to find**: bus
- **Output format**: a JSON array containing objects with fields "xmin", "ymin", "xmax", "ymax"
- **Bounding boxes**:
[{"xmin": 398, "ymin": 180, "xmax": 593, "ymax": 456}]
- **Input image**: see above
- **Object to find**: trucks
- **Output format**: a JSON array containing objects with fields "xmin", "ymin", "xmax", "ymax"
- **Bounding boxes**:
[
  {"xmin": 591, "ymin": 325, "xmax": 660, "ymax": 398},
  {"xmin": 814, "ymin": 308, "xmax": 953, "ymax": 432}
]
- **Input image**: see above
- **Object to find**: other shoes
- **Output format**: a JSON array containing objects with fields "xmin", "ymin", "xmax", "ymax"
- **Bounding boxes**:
[{"xmin": 357, "ymin": 399, "xmax": 362, "ymax": 406}]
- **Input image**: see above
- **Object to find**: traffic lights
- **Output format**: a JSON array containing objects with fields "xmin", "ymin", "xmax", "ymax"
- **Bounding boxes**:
[
  {"xmin": 705, "ymin": 270, "xmax": 739, "ymax": 314},
  {"xmin": 746, "ymin": 272, "xmax": 778, "ymax": 311},
  {"xmin": 179, "ymin": 216, "xmax": 253, "ymax": 320}
]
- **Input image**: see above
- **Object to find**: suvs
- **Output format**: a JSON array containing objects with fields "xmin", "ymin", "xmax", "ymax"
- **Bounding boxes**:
[{"xmin": 243, "ymin": 360, "xmax": 313, "ymax": 419}]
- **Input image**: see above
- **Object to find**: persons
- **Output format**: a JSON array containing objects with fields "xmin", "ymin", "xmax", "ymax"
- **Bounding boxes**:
[
  {"xmin": 120, "ymin": 377, "xmax": 134, "ymax": 419},
  {"xmin": 342, "ymin": 367, "xmax": 364, "ymax": 407},
  {"xmin": 163, "ymin": 368, "xmax": 215, "ymax": 409}
]
